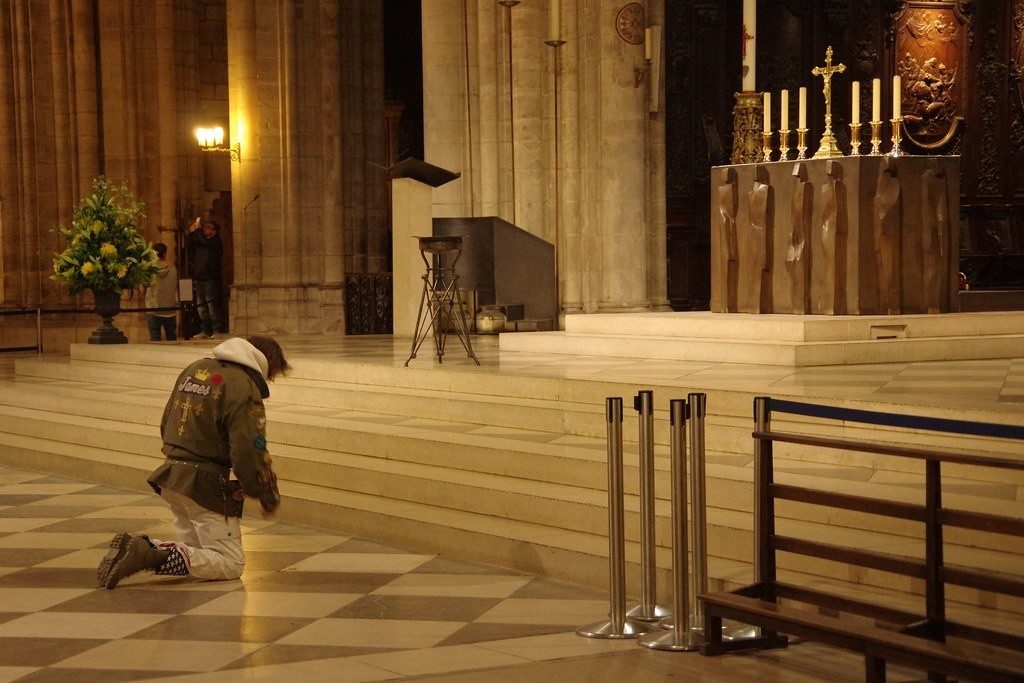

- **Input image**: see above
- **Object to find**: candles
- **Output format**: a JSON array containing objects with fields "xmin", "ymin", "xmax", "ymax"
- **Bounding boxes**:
[
  {"xmin": 781, "ymin": 90, "xmax": 789, "ymax": 130},
  {"xmin": 872, "ymin": 78, "xmax": 881, "ymax": 122},
  {"xmin": 764, "ymin": 92, "xmax": 771, "ymax": 132},
  {"xmin": 799, "ymin": 87, "xmax": 806, "ymax": 129},
  {"xmin": 852, "ymin": 81, "xmax": 860, "ymax": 124},
  {"xmin": 893, "ymin": 76, "xmax": 901, "ymax": 120}
]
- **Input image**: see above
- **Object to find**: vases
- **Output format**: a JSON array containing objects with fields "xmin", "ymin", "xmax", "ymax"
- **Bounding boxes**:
[{"xmin": 85, "ymin": 286, "xmax": 128, "ymax": 344}]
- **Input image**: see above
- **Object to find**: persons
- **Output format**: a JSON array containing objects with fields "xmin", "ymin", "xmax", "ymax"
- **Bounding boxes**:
[
  {"xmin": 185, "ymin": 220, "xmax": 225, "ymax": 340},
  {"xmin": 96, "ymin": 333, "xmax": 288, "ymax": 592},
  {"xmin": 145, "ymin": 243, "xmax": 180, "ymax": 341}
]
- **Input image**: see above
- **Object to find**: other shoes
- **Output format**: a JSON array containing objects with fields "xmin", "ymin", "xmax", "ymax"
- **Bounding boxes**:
[{"xmin": 193, "ymin": 331, "xmax": 215, "ymax": 339}]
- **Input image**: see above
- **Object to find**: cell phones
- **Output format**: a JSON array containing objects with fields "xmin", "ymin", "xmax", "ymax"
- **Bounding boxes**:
[{"xmin": 195, "ymin": 217, "xmax": 201, "ymax": 226}]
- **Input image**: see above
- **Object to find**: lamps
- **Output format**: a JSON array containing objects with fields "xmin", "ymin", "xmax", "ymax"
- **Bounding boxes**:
[{"xmin": 194, "ymin": 127, "xmax": 241, "ymax": 163}]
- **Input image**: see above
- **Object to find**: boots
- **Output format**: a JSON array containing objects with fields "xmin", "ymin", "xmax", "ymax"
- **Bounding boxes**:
[
  {"xmin": 96, "ymin": 532, "xmax": 132, "ymax": 587},
  {"xmin": 105, "ymin": 535, "xmax": 191, "ymax": 590}
]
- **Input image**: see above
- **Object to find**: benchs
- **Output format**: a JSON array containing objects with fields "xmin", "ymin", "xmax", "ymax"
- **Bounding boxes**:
[{"xmin": 697, "ymin": 432, "xmax": 1024, "ymax": 683}]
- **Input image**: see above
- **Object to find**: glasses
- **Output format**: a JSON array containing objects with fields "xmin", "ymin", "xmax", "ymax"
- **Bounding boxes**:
[{"xmin": 202, "ymin": 225, "xmax": 213, "ymax": 230}]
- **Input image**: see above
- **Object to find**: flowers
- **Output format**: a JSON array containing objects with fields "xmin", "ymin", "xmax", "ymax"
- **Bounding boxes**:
[{"xmin": 46, "ymin": 175, "xmax": 160, "ymax": 300}]
